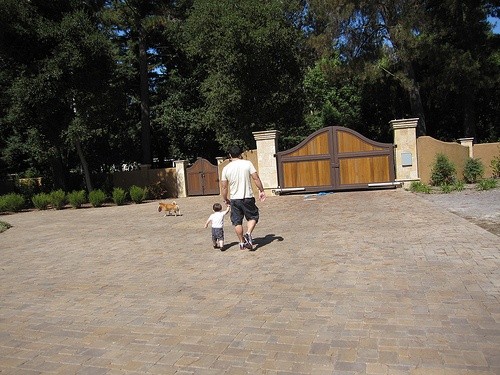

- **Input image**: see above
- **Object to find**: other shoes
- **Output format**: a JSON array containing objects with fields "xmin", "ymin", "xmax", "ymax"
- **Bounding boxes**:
[
  {"xmin": 220, "ymin": 247, "xmax": 225, "ymax": 251},
  {"xmin": 213, "ymin": 244, "xmax": 218, "ymax": 249}
]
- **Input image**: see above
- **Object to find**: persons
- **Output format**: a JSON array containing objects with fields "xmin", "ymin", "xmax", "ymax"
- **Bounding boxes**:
[
  {"xmin": 202, "ymin": 200, "xmax": 231, "ymax": 251},
  {"xmin": 219, "ymin": 146, "xmax": 266, "ymax": 250}
]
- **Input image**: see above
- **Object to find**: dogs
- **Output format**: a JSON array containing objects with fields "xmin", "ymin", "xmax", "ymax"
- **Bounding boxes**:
[{"xmin": 159, "ymin": 201, "xmax": 180, "ymax": 217}]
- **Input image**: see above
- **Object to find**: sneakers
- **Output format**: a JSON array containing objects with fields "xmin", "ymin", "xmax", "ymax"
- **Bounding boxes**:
[
  {"xmin": 239, "ymin": 242, "xmax": 245, "ymax": 250},
  {"xmin": 243, "ymin": 233, "xmax": 252, "ymax": 249}
]
